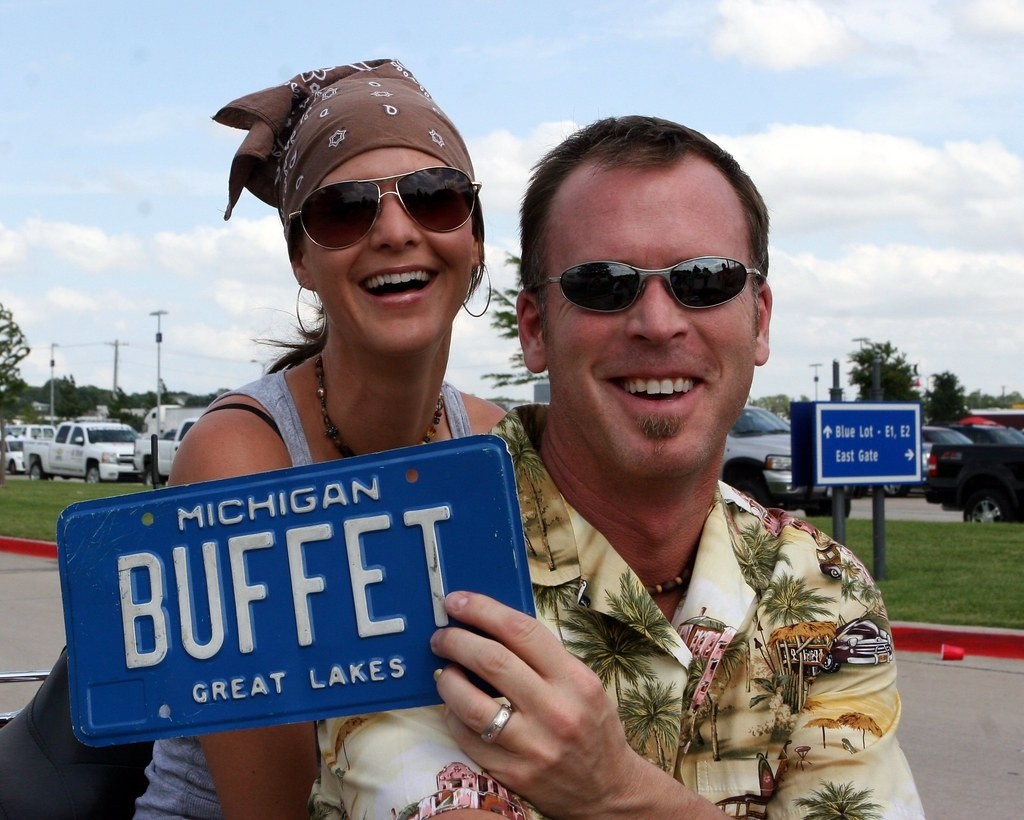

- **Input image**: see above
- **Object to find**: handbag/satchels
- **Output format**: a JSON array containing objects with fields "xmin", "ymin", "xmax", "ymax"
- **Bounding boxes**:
[{"xmin": 0, "ymin": 637, "xmax": 151, "ymax": 820}]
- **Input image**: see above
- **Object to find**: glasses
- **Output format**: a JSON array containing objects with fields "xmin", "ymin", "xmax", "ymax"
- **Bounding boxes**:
[
  {"xmin": 524, "ymin": 255, "xmax": 765, "ymax": 313},
  {"xmin": 291, "ymin": 166, "xmax": 482, "ymax": 250}
]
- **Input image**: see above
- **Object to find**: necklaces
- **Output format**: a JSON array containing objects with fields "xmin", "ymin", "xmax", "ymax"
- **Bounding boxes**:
[
  {"xmin": 644, "ymin": 548, "xmax": 697, "ymax": 596},
  {"xmin": 313, "ymin": 350, "xmax": 447, "ymax": 458}
]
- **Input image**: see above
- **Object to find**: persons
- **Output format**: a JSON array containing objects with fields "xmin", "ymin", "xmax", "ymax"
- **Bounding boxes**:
[{"xmin": 134, "ymin": 59, "xmax": 925, "ymax": 820}]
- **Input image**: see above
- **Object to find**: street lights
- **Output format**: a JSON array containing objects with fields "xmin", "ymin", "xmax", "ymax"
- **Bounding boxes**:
[{"xmin": 150, "ymin": 310, "xmax": 170, "ymax": 436}]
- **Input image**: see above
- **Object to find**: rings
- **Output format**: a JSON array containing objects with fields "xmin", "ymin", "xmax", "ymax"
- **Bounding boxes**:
[{"xmin": 481, "ymin": 704, "xmax": 512, "ymax": 744}]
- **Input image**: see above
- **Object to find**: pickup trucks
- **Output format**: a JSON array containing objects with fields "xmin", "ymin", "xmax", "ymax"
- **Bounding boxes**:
[{"xmin": 924, "ymin": 442, "xmax": 1024, "ymax": 523}]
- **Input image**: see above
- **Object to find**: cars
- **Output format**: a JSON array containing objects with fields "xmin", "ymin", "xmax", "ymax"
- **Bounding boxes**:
[
  {"xmin": 718, "ymin": 404, "xmax": 855, "ymax": 521},
  {"xmin": 4, "ymin": 402, "xmax": 209, "ymax": 485},
  {"xmin": 881, "ymin": 427, "xmax": 975, "ymax": 499},
  {"xmin": 948, "ymin": 424, "xmax": 1024, "ymax": 445}
]
